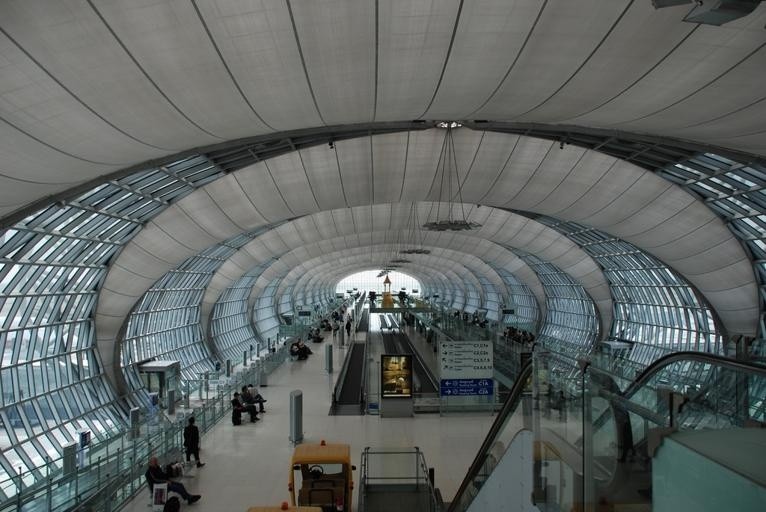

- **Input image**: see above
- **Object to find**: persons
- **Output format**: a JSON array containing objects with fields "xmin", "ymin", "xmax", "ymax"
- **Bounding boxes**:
[
  {"xmin": 454, "ymin": 310, "xmax": 488, "ymax": 328},
  {"xmin": 231, "ymin": 392, "xmax": 260, "ymax": 423},
  {"xmin": 542, "ymin": 384, "xmax": 553, "ymax": 419},
  {"xmin": 248, "ymin": 384, "xmax": 258, "ymax": 397},
  {"xmin": 241, "ymin": 385, "xmax": 267, "ymax": 412},
  {"xmin": 290, "ymin": 309, "xmax": 351, "ymax": 360},
  {"xmin": 163, "ymin": 496, "xmax": 181, "ymax": 512},
  {"xmin": 145, "ymin": 457, "xmax": 201, "ymax": 506},
  {"xmin": 558, "ymin": 391, "xmax": 567, "ymax": 422},
  {"xmin": 183, "ymin": 417, "xmax": 205, "ymax": 468},
  {"xmin": 504, "ymin": 326, "xmax": 535, "ymax": 344}
]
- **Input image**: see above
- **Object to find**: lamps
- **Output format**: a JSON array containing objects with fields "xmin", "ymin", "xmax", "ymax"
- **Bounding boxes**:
[
  {"xmin": 421, "ymin": 123, "xmax": 483, "ymax": 232},
  {"xmin": 398, "ymin": 199, "xmax": 431, "ymax": 254},
  {"xmin": 376, "ymin": 226, "xmax": 412, "ymax": 278}
]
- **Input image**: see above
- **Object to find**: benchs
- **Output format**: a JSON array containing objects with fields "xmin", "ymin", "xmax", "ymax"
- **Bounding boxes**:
[
  {"xmin": 145, "ymin": 447, "xmax": 201, "ymax": 507},
  {"xmin": 229, "ymin": 395, "xmax": 252, "ymax": 414},
  {"xmin": 297, "ymin": 470, "xmax": 346, "ymax": 509}
]
